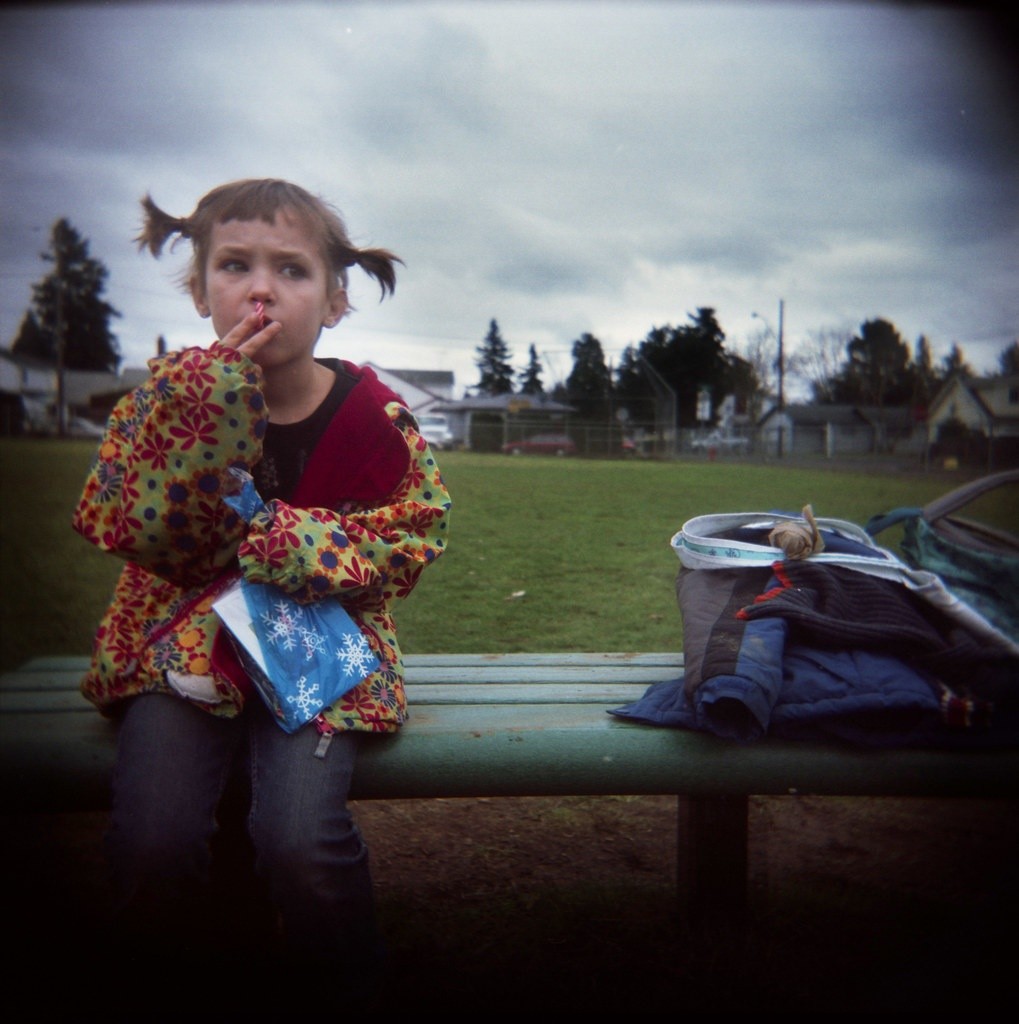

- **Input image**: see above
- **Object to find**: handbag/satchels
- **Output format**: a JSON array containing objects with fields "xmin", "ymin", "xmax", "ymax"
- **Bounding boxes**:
[{"xmin": 670, "ymin": 468, "xmax": 1019, "ymax": 660}]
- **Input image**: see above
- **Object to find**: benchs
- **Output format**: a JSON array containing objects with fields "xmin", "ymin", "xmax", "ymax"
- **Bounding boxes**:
[{"xmin": 0, "ymin": 654, "xmax": 1019, "ymax": 937}]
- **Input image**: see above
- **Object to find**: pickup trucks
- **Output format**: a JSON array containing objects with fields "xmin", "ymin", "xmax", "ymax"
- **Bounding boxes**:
[{"xmin": 690, "ymin": 429, "xmax": 750, "ymax": 456}]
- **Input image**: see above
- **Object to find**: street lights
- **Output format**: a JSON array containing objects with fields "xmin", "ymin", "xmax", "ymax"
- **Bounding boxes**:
[{"xmin": 751, "ymin": 311, "xmax": 783, "ymax": 461}]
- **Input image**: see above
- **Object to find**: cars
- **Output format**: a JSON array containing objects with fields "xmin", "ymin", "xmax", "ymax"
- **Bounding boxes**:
[
  {"xmin": 70, "ymin": 417, "xmax": 105, "ymax": 438},
  {"xmin": 502, "ymin": 433, "xmax": 577, "ymax": 457},
  {"xmin": 415, "ymin": 414, "xmax": 454, "ymax": 451}
]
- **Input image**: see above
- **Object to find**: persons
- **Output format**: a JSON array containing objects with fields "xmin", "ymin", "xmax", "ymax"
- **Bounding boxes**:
[{"xmin": 71, "ymin": 182, "xmax": 454, "ymax": 1023}]
